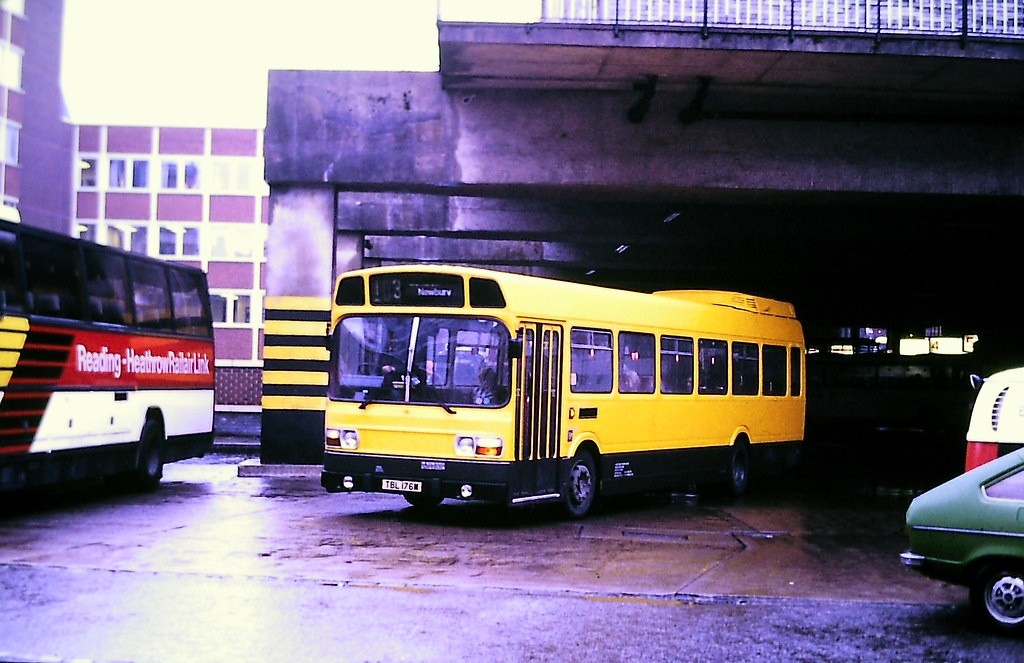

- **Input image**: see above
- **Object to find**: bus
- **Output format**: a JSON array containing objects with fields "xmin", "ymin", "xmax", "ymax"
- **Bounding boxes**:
[
  {"xmin": 317, "ymin": 262, "xmax": 807, "ymax": 521},
  {"xmin": 0, "ymin": 217, "xmax": 215, "ymax": 507}
]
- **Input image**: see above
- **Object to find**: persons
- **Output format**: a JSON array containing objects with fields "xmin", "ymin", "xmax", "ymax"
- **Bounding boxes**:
[
  {"xmin": 623, "ymin": 371, "xmax": 642, "ymax": 394},
  {"xmin": 685, "ymin": 376, "xmax": 694, "ymax": 394},
  {"xmin": 469, "ymin": 345, "xmax": 486, "ymax": 383},
  {"xmin": 473, "ymin": 367, "xmax": 509, "ymax": 408}
]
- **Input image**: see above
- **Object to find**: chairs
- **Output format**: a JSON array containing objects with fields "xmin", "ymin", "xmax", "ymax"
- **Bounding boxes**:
[{"xmin": 544, "ymin": 368, "xmax": 798, "ymax": 402}]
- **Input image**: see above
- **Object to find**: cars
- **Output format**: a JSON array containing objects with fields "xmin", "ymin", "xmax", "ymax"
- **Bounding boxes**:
[{"xmin": 905, "ymin": 444, "xmax": 1024, "ymax": 637}]
